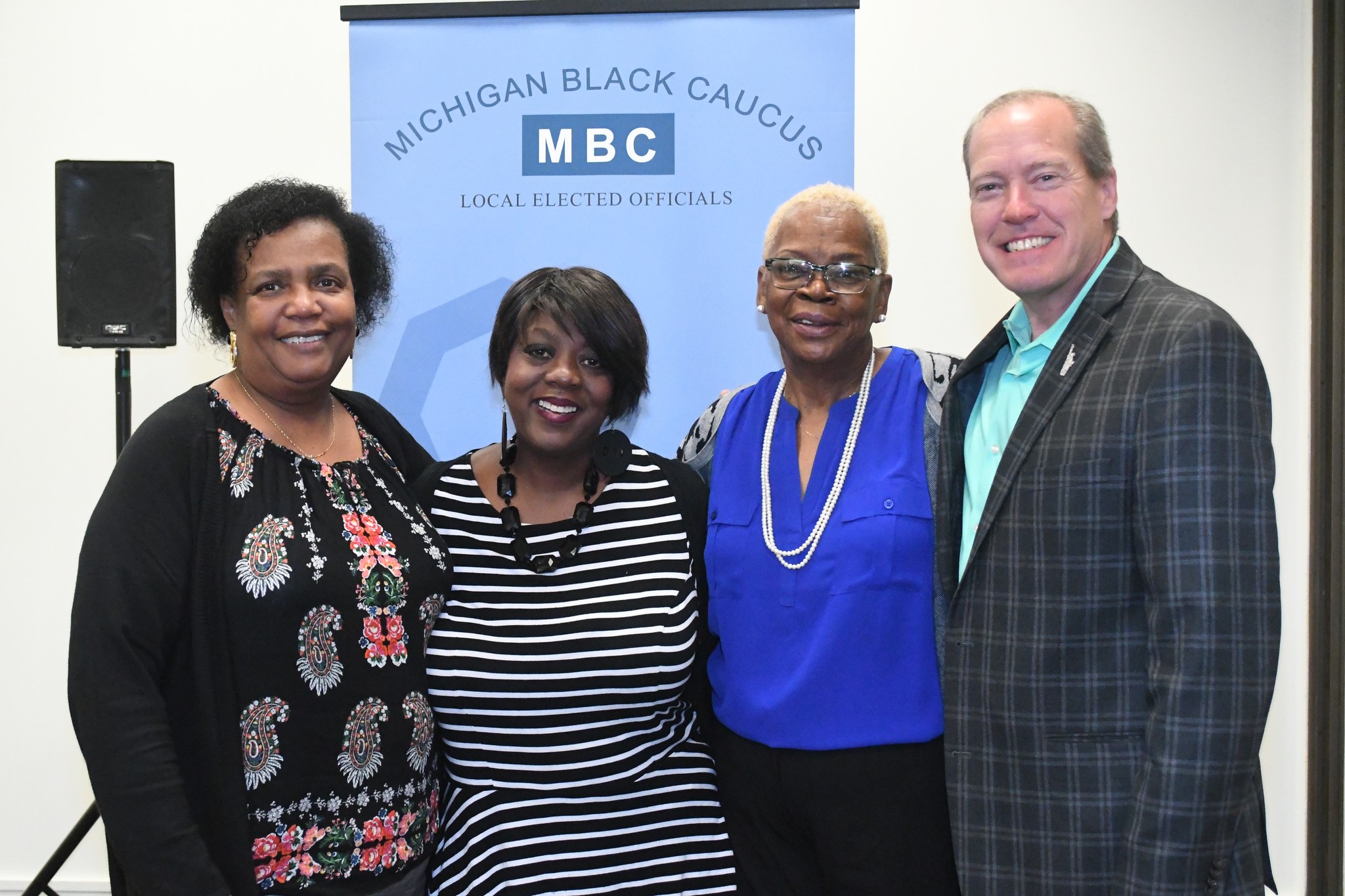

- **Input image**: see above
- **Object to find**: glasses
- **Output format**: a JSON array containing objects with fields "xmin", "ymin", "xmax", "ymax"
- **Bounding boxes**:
[{"xmin": 765, "ymin": 258, "xmax": 883, "ymax": 294}]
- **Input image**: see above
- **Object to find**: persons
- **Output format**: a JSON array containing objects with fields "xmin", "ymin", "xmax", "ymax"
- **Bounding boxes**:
[
  {"xmin": 677, "ymin": 181, "xmax": 968, "ymax": 894},
  {"xmin": 415, "ymin": 264, "xmax": 739, "ymax": 894},
  {"xmin": 71, "ymin": 179, "xmax": 452, "ymax": 896},
  {"xmin": 938, "ymin": 90, "xmax": 1286, "ymax": 894}
]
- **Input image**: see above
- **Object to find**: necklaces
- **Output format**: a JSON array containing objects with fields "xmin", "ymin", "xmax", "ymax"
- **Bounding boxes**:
[
  {"xmin": 761, "ymin": 347, "xmax": 875, "ymax": 569},
  {"xmin": 496, "ymin": 433, "xmax": 601, "ymax": 573},
  {"xmin": 233, "ymin": 369, "xmax": 335, "ymax": 458}
]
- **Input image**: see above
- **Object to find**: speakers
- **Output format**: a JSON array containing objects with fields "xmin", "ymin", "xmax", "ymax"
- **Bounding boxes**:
[{"xmin": 53, "ymin": 159, "xmax": 180, "ymax": 351}]
